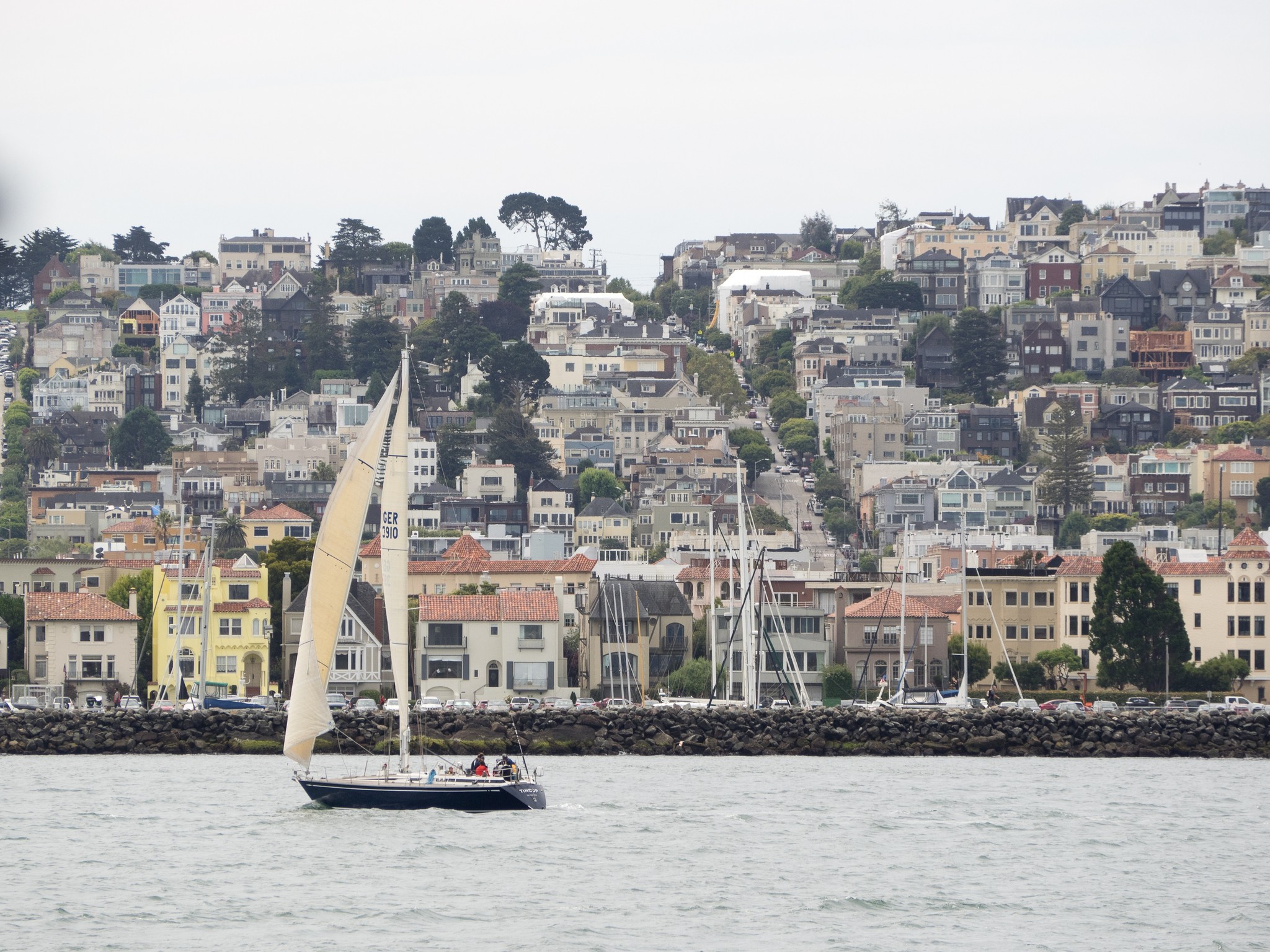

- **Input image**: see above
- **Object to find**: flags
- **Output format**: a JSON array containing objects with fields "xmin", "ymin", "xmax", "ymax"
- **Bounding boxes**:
[{"xmin": 63, "ymin": 664, "xmax": 67, "ymax": 679}]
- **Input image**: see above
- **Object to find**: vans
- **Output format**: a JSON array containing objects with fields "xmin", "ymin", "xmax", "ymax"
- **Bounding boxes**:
[
  {"xmin": 666, "ymin": 316, "xmax": 676, "ymax": 326},
  {"xmin": 697, "ymin": 343, "xmax": 715, "ymax": 356}
]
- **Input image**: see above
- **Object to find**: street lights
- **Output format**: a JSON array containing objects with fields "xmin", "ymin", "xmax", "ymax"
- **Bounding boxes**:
[
  {"xmin": 754, "ymin": 458, "xmax": 769, "ymax": 492},
  {"xmin": 719, "ymin": 392, "xmax": 734, "ymax": 415}
]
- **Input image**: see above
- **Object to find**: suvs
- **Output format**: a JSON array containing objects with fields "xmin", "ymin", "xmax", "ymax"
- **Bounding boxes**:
[
  {"xmin": 748, "ymin": 409, "xmax": 757, "ymax": 418},
  {"xmin": 801, "ymin": 520, "xmax": 812, "ymax": 531},
  {"xmin": 777, "ymin": 442, "xmax": 786, "ymax": 451},
  {"xmin": 781, "ymin": 449, "xmax": 793, "ymax": 458},
  {"xmin": 764, "ymin": 437, "xmax": 770, "ymax": 445},
  {"xmin": 752, "ymin": 421, "xmax": 763, "ymax": 430},
  {"xmin": 787, "ymin": 462, "xmax": 800, "ymax": 473},
  {"xmin": 784, "ymin": 456, "xmax": 796, "ymax": 466},
  {"xmin": 779, "ymin": 466, "xmax": 791, "ymax": 474}
]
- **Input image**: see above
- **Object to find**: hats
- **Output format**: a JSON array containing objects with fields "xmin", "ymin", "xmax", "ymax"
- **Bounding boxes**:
[{"xmin": 502, "ymin": 754, "xmax": 507, "ymax": 758}]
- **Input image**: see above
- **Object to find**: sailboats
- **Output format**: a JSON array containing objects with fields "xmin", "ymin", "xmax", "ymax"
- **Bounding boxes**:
[
  {"xmin": 282, "ymin": 332, "xmax": 545, "ymax": 813},
  {"xmin": 850, "ymin": 529, "xmax": 1029, "ymax": 713},
  {"xmin": 599, "ymin": 457, "xmax": 824, "ymax": 709},
  {"xmin": 122, "ymin": 502, "xmax": 270, "ymax": 712}
]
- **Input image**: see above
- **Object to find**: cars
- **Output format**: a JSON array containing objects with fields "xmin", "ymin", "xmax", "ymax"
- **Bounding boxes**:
[
  {"xmin": 765, "ymin": 412, "xmax": 779, "ymax": 432},
  {"xmin": 967, "ymin": 695, "xmax": 1266, "ymax": 715},
  {"xmin": 799, "ymin": 466, "xmax": 861, "ymax": 572},
  {"xmin": 672, "ymin": 326, "xmax": 694, "ymax": 346},
  {"xmin": 737, "ymin": 374, "xmax": 764, "ymax": 410},
  {"xmin": 0, "ymin": 692, "xmax": 872, "ymax": 712},
  {"xmin": 774, "ymin": 465, "xmax": 783, "ymax": 473}
]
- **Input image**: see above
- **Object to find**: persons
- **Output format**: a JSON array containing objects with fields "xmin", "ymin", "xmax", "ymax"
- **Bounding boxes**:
[
  {"xmin": 471, "ymin": 752, "xmax": 490, "ymax": 777},
  {"xmin": 380, "ymin": 695, "xmax": 386, "ymax": 705},
  {"xmin": 495, "ymin": 754, "xmax": 518, "ymax": 782},
  {"xmin": 987, "ymin": 686, "xmax": 1000, "ymax": 708},
  {"xmin": 113, "ymin": 688, "xmax": 121, "ymax": 708},
  {"xmin": 347, "ymin": 701, "xmax": 353, "ymax": 711},
  {"xmin": 645, "ymin": 693, "xmax": 651, "ymax": 700}
]
